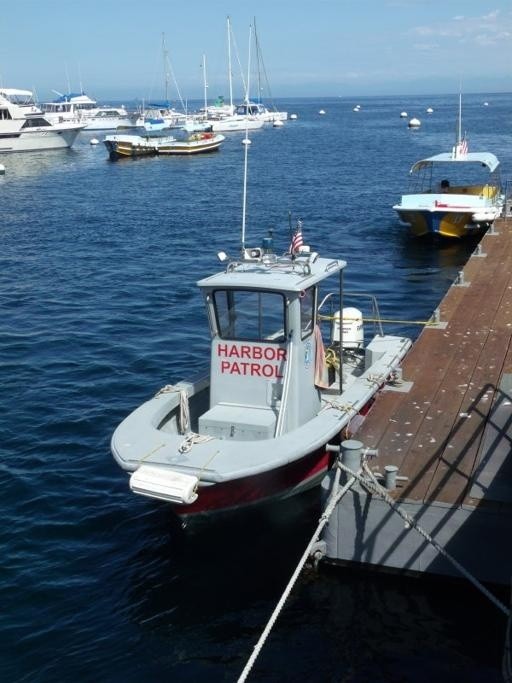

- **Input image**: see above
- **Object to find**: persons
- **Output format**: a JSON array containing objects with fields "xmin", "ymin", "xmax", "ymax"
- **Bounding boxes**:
[{"xmin": 441, "ymin": 180, "xmax": 449, "ymax": 193}]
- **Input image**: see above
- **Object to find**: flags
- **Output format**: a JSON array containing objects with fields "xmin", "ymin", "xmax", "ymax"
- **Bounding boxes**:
[
  {"xmin": 460, "ymin": 138, "xmax": 468, "ymax": 153},
  {"xmin": 290, "ymin": 221, "xmax": 303, "ymax": 255}
]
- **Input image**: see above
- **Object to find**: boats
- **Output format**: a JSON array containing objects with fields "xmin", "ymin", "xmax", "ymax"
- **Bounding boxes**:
[
  {"xmin": 110, "ymin": 129, "xmax": 413, "ymax": 519},
  {"xmin": 38, "ymin": 15, "xmax": 288, "ymax": 132},
  {"xmin": 0, "ymin": 88, "xmax": 88, "ymax": 152},
  {"xmin": 102, "ymin": 133, "xmax": 226, "ymax": 160},
  {"xmin": 392, "ymin": 96, "xmax": 505, "ymax": 239}
]
